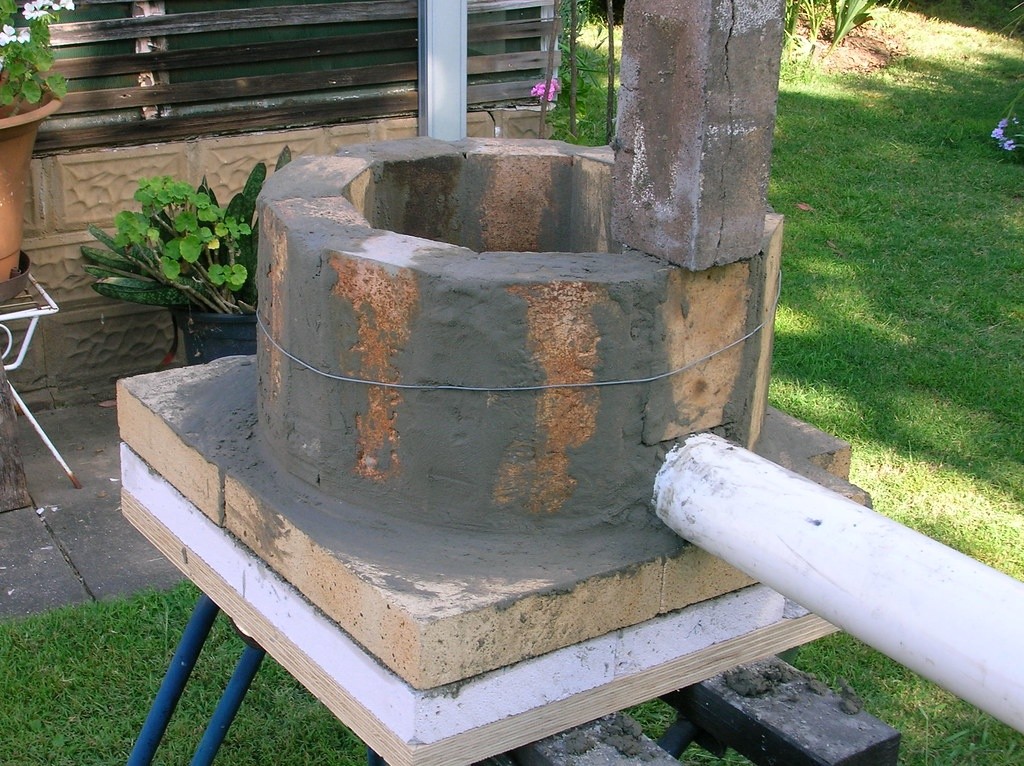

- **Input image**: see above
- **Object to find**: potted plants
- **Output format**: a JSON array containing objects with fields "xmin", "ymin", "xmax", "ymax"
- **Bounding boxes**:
[{"xmin": 79, "ymin": 143, "xmax": 292, "ymax": 364}]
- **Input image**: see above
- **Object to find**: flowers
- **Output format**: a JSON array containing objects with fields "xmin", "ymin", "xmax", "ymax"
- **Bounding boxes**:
[{"xmin": 0, "ymin": 1, "xmax": 71, "ymax": 114}]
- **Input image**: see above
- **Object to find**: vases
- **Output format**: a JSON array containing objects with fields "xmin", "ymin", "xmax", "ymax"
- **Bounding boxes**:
[{"xmin": 0, "ymin": 77, "xmax": 65, "ymax": 281}]
